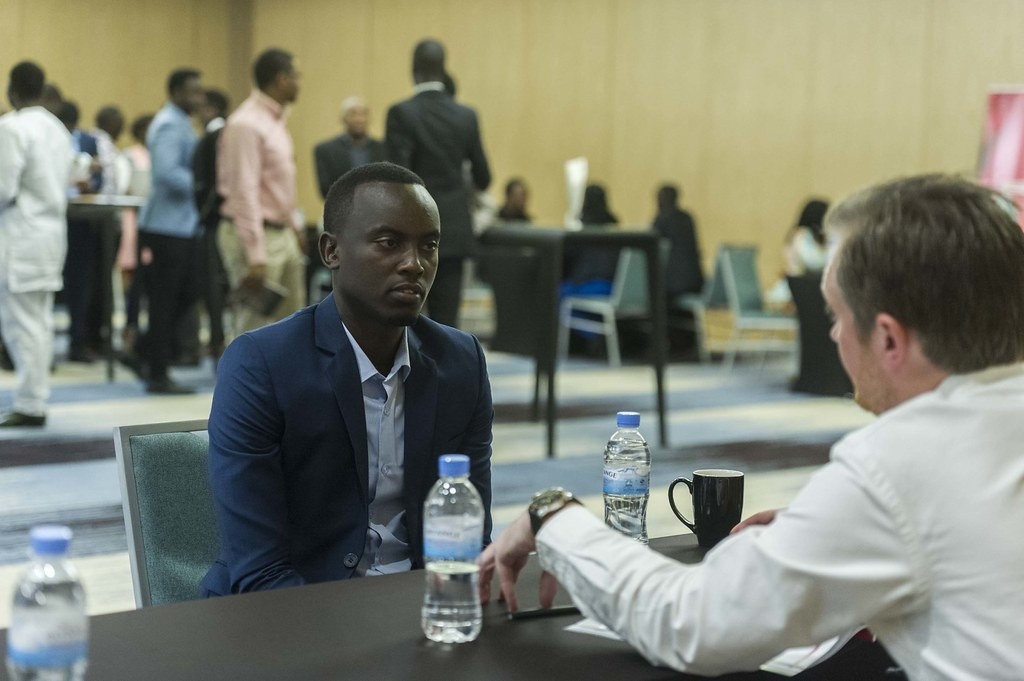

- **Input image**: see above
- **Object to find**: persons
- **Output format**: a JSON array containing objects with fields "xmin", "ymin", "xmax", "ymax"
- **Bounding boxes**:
[
  {"xmin": 117, "ymin": 67, "xmax": 229, "ymax": 394},
  {"xmin": 495, "ymin": 180, "xmax": 531, "ymax": 220},
  {"xmin": 650, "ymin": 183, "xmax": 704, "ymax": 302},
  {"xmin": 312, "ymin": 95, "xmax": 393, "ymax": 200},
  {"xmin": 214, "ymin": 47, "xmax": 310, "ymax": 344},
  {"xmin": 474, "ymin": 159, "xmax": 1022, "ymax": 681},
  {"xmin": 779, "ymin": 201, "xmax": 830, "ymax": 279},
  {"xmin": 199, "ymin": 161, "xmax": 499, "ymax": 598},
  {"xmin": 386, "ymin": 38, "xmax": 491, "ymax": 333},
  {"xmin": 581, "ymin": 185, "xmax": 621, "ymax": 225},
  {"xmin": 0, "ymin": 57, "xmax": 118, "ymax": 431}
]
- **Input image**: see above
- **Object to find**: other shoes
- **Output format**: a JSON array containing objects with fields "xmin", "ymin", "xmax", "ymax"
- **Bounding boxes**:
[
  {"xmin": 120, "ymin": 353, "xmax": 151, "ymax": 382},
  {"xmin": 147, "ymin": 378, "xmax": 196, "ymax": 394},
  {"xmin": 0, "ymin": 411, "xmax": 44, "ymax": 427}
]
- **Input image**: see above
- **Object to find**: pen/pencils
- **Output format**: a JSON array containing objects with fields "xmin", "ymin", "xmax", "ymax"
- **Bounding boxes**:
[{"xmin": 507, "ymin": 605, "xmax": 581, "ymax": 621}]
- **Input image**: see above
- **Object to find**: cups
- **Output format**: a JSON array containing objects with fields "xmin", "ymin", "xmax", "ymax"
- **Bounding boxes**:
[{"xmin": 666, "ymin": 467, "xmax": 744, "ymax": 549}]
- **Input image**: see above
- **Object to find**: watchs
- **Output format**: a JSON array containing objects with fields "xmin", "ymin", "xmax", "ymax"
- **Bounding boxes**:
[{"xmin": 528, "ymin": 487, "xmax": 586, "ymax": 536}]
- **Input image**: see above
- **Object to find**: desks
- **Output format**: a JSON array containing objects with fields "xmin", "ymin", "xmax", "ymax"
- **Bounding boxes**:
[
  {"xmin": 0, "ymin": 532, "xmax": 911, "ymax": 681},
  {"xmin": 787, "ymin": 274, "xmax": 853, "ymax": 393}
]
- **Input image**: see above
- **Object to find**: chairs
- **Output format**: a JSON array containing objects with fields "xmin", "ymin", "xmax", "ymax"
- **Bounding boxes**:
[
  {"xmin": 563, "ymin": 245, "xmax": 652, "ymax": 364},
  {"xmin": 717, "ymin": 253, "xmax": 800, "ymax": 372},
  {"xmin": 116, "ymin": 420, "xmax": 223, "ymax": 608},
  {"xmin": 659, "ymin": 247, "xmax": 746, "ymax": 364}
]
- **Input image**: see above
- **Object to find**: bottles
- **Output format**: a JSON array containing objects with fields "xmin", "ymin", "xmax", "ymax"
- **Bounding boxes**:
[
  {"xmin": 4, "ymin": 523, "xmax": 91, "ymax": 681},
  {"xmin": 420, "ymin": 454, "xmax": 484, "ymax": 645},
  {"xmin": 602, "ymin": 411, "xmax": 651, "ymax": 548}
]
